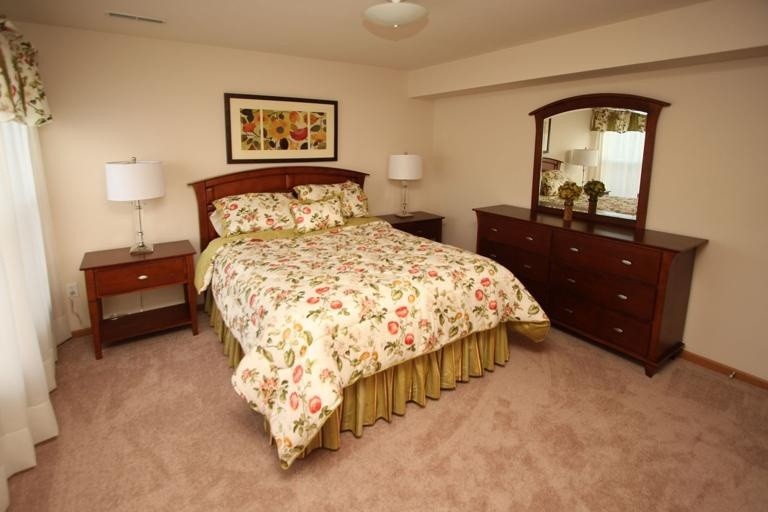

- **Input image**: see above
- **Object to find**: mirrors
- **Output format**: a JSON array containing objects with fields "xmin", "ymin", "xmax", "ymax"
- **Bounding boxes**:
[{"xmin": 528, "ymin": 93, "xmax": 671, "ymax": 229}]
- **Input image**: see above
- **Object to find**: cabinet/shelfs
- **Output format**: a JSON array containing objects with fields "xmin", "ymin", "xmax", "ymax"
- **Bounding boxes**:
[{"xmin": 472, "ymin": 204, "xmax": 708, "ymax": 377}]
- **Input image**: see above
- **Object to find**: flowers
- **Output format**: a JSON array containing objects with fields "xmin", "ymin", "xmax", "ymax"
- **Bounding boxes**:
[
  {"xmin": 558, "ymin": 181, "xmax": 582, "ymax": 199},
  {"xmin": 584, "ymin": 181, "xmax": 606, "ymax": 197}
]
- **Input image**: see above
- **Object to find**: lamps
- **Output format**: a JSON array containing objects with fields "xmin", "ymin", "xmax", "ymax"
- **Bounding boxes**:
[
  {"xmin": 571, "ymin": 147, "xmax": 598, "ymax": 184},
  {"xmin": 106, "ymin": 158, "xmax": 165, "ymax": 254},
  {"xmin": 388, "ymin": 153, "xmax": 423, "ymax": 218}
]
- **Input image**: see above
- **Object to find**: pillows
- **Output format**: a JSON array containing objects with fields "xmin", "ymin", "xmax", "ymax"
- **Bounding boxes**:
[
  {"xmin": 212, "ymin": 193, "xmax": 298, "ymax": 238},
  {"xmin": 541, "ymin": 169, "xmax": 574, "ymax": 196},
  {"xmin": 290, "ymin": 195, "xmax": 345, "ymax": 234},
  {"xmin": 293, "ymin": 182, "xmax": 370, "ymax": 219}
]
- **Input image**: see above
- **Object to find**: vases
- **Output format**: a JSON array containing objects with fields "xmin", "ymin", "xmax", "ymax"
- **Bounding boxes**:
[
  {"xmin": 588, "ymin": 199, "xmax": 596, "ymax": 214},
  {"xmin": 564, "ymin": 203, "xmax": 573, "ymax": 221}
]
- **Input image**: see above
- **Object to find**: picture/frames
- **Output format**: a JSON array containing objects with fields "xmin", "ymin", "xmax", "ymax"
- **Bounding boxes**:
[
  {"xmin": 542, "ymin": 118, "xmax": 550, "ymax": 152},
  {"xmin": 224, "ymin": 93, "xmax": 338, "ymax": 164}
]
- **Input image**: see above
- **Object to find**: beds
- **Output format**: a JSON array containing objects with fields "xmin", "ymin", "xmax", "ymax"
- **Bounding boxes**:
[
  {"xmin": 188, "ymin": 167, "xmax": 550, "ymax": 469},
  {"xmin": 539, "ymin": 158, "xmax": 638, "ymax": 220}
]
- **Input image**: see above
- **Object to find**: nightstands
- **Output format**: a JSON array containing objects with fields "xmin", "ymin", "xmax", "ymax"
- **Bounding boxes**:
[
  {"xmin": 377, "ymin": 210, "xmax": 446, "ymax": 243},
  {"xmin": 80, "ymin": 240, "xmax": 198, "ymax": 359}
]
